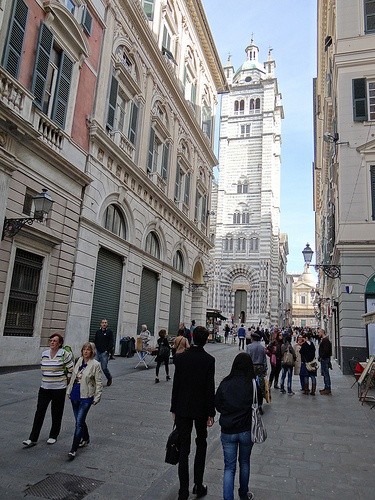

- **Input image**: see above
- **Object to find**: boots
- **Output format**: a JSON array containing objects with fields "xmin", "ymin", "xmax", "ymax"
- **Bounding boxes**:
[
  {"xmin": 303, "ymin": 383, "xmax": 309, "ymax": 395},
  {"xmin": 309, "ymin": 383, "xmax": 316, "ymax": 396}
]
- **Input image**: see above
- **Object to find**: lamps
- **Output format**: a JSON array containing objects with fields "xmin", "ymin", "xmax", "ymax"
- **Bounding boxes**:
[
  {"xmin": 188, "ymin": 272, "xmax": 209, "ymax": 293},
  {"xmin": 1, "ymin": 189, "xmax": 55, "ymax": 241},
  {"xmin": 302, "ymin": 242, "xmax": 341, "ymax": 280},
  {"xmin": 311, "ymin": 288, "xmax": 315, "ymax": 299}
]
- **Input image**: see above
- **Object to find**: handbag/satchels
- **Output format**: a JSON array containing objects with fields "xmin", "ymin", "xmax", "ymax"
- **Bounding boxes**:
[
  {"xmin": 172, "ymin": 348, "xmax": 176, "ymax": 356},
  {"xmin": 305, "ymin": 358, "xmax": 319, "ymax": 371},
  {"xmin": 62, "ymin": 346, "xmax": 75, "ymax": 375},
  {"xmin": 137, "ymin": 335, "xmax": 144, "ymax": 351},
  {"xmin": 159, "ymin": 344, "xmax": 170, "ymax": 357},
  {"xmin": 265, "ymin": 340, "xmax": 277, "ymax": 355},
  {"xmin": 264, "ymin": 375, "xmax": 272, "ymax": 403},
  {"xmin": 252, "ymin": 379, "xmax": 267, "ymax": 444},
  {"xmin": 165, "ymin": 429, "xmax": 180, "ymax": 465},
  {"xmin": 281, "ymin": 352, "xmax": 295, "ymax": 366}
]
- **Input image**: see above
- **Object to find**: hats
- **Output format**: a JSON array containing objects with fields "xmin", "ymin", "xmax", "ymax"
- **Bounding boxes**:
[{"xmin": 251, "ymin": 330, "xmax": 262, "ymax": 341}]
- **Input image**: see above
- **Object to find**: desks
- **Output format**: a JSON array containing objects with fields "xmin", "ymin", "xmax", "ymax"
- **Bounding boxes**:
[{"xmin": 135, "ymin": 349, "xmax": 158, "ymax": 368}]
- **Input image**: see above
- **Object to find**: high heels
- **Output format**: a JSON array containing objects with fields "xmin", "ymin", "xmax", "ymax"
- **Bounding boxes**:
[
  {"xmin": 166, "ymin": 375, "xmax": 172, "ymax": 381},
  {"xmin": 155, "ymin": 378, "xmax": 160, "ymax": 383}
]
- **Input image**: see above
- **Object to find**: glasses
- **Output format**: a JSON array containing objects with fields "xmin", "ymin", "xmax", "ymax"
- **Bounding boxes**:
[{"xmin": 49, "ymin": 339, "xmax": 57, "ymax": 342}]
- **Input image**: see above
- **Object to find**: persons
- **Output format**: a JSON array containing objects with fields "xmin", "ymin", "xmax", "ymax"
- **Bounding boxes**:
[
  {"xmin": 266, "ymin": 332, "xmax": 284, "ymax": 389},
  {"xmin": 293, "ymin": 334, "xmax": 306, "ymax": 391},
  {"xmin": 215, "ymin": 353, "xmax": 262, "ymax": 500},
  {"xmin": 94, "ymin": 319, "xmax": 115, "ymax": 386},
  {"xmin": 230, "ymin": 325, "xmax": 237, "ymax": 342},
  {"xmin": 247, "ymin": 325, "xmax": 320, "ymax": 346},
  {"xmin": 317, "ymin": 329, "xmax": 332, "ymax": 396},
  {"xmin": 139, "ymin": 324, "xmax": 150, "ymax": 361},
  {"xmin": 66, "ymin": 343, "xmax": 103, "ymax": 458},
  {"xmin": 247, "ymin": 333, "xmax": 268, "ymax": 416},
  {"xmin": 238, "ymin": 324, "xmax": 246, "ymax": 350},
  {"xmin": 189, "ymin": 320, "xmax": 197, "ymax": 346},
  {"xmin": 155, "ymin": 329, "xmax": 172, "ymax": 383},
  {"xmin": 298, "ymin": 333, "xmax": 316, "ymax": 396},
  {"xmin": 280, "ymin": 335, "xmax": 296, "ymax": 395},
  {"xmin": 174, "ymin": 328, "xmax": 190, "ymax": 353},
  {"xmin": 23, "ymin": 334, "xmax": 74, "ymax": 446},
  {"xmin": 211, "ymin": 328, "xmax": 217, "ymax": 338},
  {"xmin": 223, "ymin": 324, "xmax": 230, "ymax": 344},
  {"xmin": 178, "ymin": 322, "xmax": 192, "ymax": 344},
  {"xmin": 170, "ymin": 327, "xmax": 216, "ymax": 500}
]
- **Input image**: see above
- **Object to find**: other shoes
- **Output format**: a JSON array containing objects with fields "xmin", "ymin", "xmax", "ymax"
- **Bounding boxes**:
[
  {"xmin": 280, "ymin": 390, "xmax": 286, "ymax": 393},
  {"xmin": 319, "ymin": 388, "xmax": 331, "ymax": 396},
  {"xmin": 23, "ymin": 440, "xmax": 37, "ymax": 446},
  {"xmin": 106, "ymin": 375, "xmax": 112, "ymax": 386},
  {"xmin": 246, "ymin": 492, "xmax": 254, "ymax": 500},
  {"xmin": 47, "ymin": 438, "xmax": 57, "ymax": 444},
  {"xmin": 288, "ymin": 391, "xmax": 295, "ymax": 395},
  {"xmin": 192, "ymin": 484, "xmax": 208, "ymax": 495},
  {"xmin": 79, "ymin": 438, "xmax": 90, "ymax": 447},
  {"xmin": 68, "ymin": 451, "xmax": 76, "ymax": 457},
  {"xmin": 274, "ymin": 385, "xmax": 280, "ymax": 389}
]
